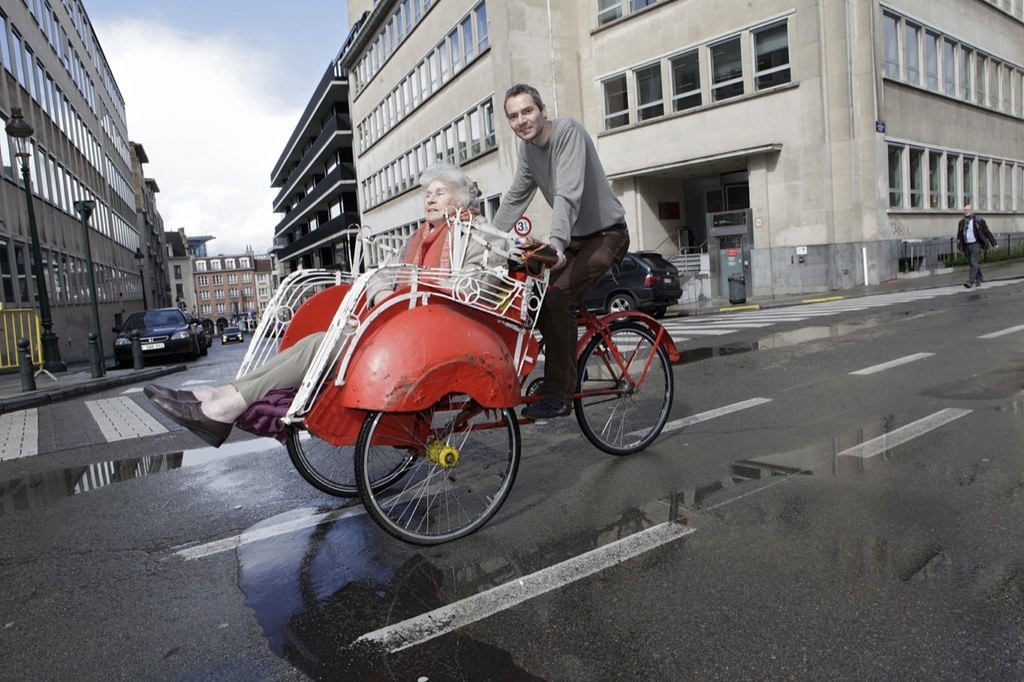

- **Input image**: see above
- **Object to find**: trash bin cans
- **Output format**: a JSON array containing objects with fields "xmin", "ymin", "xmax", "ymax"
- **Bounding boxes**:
[
  {"xmin": 113, "ymin": 333, "xmax": 133, "ymax": 368},
  {"xmin": 728, "ymin": 275, "xmax": 746, "ymax": 304}
]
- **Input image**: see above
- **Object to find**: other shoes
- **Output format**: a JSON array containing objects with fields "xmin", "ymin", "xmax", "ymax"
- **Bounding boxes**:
[
  {"xmin": 976, "ymin": 277, "xmax": 983, "ymax": 287},
  {"xmin": 964, "ymin": 280, "xmax": 973, "ymax": 287},
  {"xmin": 521, "ymin": 398, "xmax": 572, "ymax": 419}
]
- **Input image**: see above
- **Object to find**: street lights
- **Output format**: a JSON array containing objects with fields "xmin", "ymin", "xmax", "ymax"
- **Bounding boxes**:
[
  {"xmin": 133, "ymin": 247, "xmax": 148, "ymax": 310},
  {"xmin": 4, "ymin": 107, "xmax": 69, "ymax": 375}
]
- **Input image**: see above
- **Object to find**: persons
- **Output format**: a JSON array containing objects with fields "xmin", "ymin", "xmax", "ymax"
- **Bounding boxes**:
[
  {"xmin": 143, "ymin": 158, "xmax": 478, "ymax": 449},
  {"xmin": 957, "ymin": 205, "xmax": 999, "ymax": 288},
  {"xmin": 491, "ymin": 84, "xmax": 630, "ymax": 419}
]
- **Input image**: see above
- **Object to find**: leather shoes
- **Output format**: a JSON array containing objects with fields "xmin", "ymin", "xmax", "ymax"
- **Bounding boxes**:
[
  {"xmin": 150, "ymin": 395, "xmax": 234, "ymax": 449},
  {"xmin": 142, "ymin": 383, "xmax": 198, "ymax": 401}
]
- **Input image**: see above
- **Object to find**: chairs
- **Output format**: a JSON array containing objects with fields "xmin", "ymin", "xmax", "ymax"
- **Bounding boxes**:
[{"xmin": 236, "ymin": 271, "xmax": 544, "ymax": 446}]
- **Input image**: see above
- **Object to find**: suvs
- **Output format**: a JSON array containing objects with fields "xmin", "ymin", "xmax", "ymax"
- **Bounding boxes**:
[{"xmin": 583, "ymin": 250, "xmax": 684, "ymax": 325}]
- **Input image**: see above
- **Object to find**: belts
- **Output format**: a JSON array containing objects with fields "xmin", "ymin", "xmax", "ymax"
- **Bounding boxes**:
[{"xmin": 965, "ymin": 241, "xmax": 978, "ymax": 245}]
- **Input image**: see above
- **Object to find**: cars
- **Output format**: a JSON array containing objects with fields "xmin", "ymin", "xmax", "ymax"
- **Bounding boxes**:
[
  {"xmin": 221, "ymin": 326, "xmax": 244, "ymax": 345},
  {"xmin": 111, "ymin": 307, "xmax": 213, "ymax": 369}
]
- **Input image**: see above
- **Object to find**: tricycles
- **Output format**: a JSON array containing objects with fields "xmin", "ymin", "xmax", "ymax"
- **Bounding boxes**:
[{"xmin": 230, "ymin": 203, "xmax": 682, "ymax": 547}]
